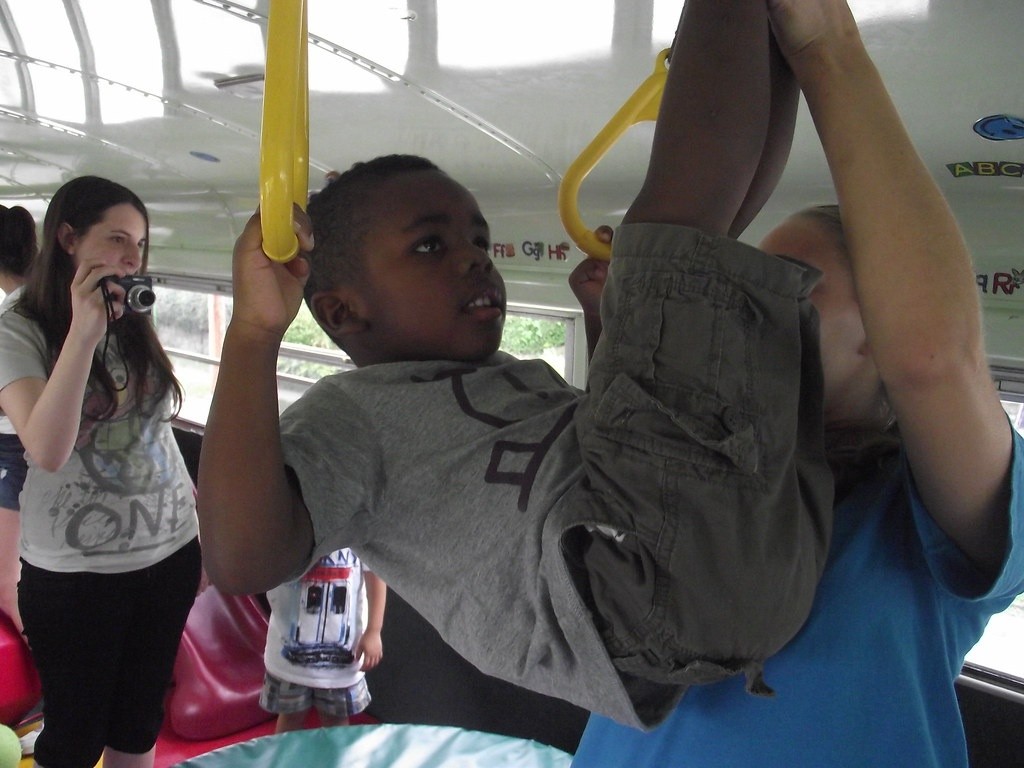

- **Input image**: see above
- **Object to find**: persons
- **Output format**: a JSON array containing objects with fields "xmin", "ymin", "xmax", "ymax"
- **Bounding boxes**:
[
  {"xmin": 0, "ymin": 176, "xmax": 203, "ymax": 768},
  {"xmin": 0, "ymin": 206, "xmax": 37, "ymax": 644},
  {"xmin": 197, "ymin": 0, "xmax": 834, "ymax": 733},
  {"xmin": 258, "ymin": 547, "xmax": 387, "ymax": 733},
  {"xmin": 570, "ymin": 0, "xmax": 1024, "ymax": 768}
]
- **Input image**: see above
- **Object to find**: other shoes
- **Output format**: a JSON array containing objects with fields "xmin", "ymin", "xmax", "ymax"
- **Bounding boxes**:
[{"xmin": 17, "ymin": 720, "xmax": 46, "ymax": 755}]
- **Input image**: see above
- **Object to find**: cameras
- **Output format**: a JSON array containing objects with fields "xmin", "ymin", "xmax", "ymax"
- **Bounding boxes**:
[{"xmin": 97, "ymin": 275, "xmax": 156, "ymax": 314}]
